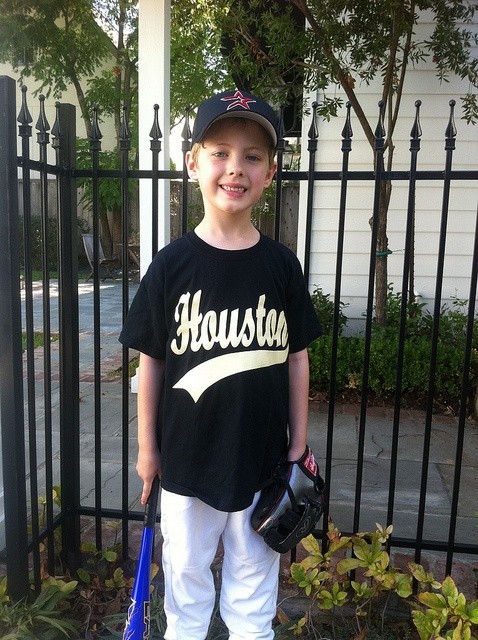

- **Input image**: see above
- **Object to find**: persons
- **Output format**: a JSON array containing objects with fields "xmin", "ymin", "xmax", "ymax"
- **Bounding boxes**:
[{"xmin": 120, "ymin": 89, "xmax": 322, "ymax": 640}]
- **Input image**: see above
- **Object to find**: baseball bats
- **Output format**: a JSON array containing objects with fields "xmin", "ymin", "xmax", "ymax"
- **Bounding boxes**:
[{"xmin": 121, "ymin": 473, "xmax": 159, "ymax": 640}]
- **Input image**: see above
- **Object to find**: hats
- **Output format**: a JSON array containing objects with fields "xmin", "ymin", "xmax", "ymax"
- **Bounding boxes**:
[{"xmin": 190, "ymin": 90, "xmax": 280, "ymax": 158}]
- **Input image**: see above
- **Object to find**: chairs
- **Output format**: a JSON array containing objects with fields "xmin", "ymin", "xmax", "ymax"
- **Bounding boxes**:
[{"xmin": 81, "ymin": 234, "xmax": 119, "ymax": 283}]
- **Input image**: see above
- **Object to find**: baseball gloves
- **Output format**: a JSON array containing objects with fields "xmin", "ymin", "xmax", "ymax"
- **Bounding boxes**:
[{"xmin": 251, "ymin": 443, "xmax": 327, "ymax": 554}]
